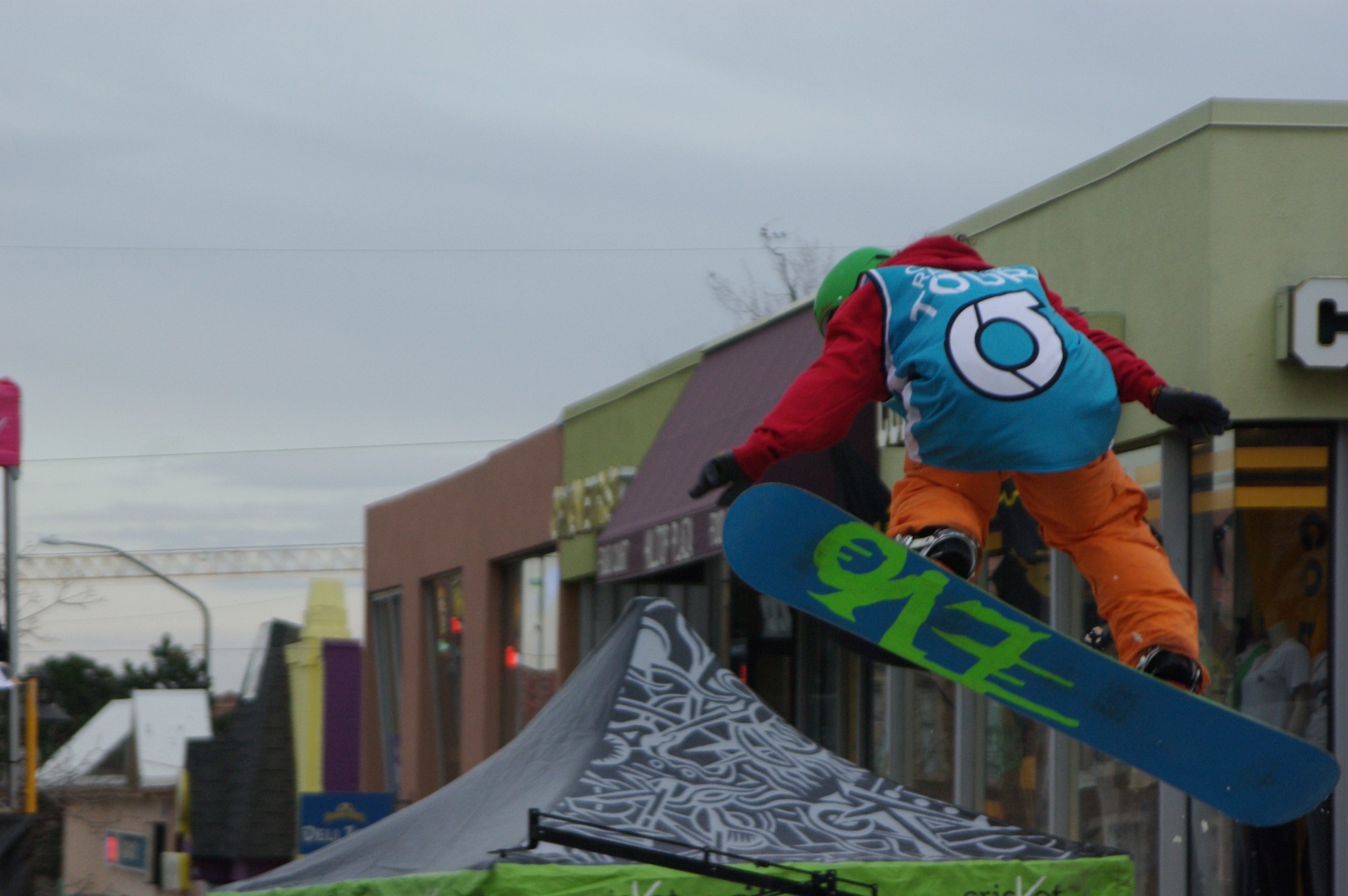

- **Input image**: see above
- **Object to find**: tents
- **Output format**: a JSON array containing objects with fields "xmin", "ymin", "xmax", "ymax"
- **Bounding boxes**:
[{"xmin": 202, "ymin": 595, "xmax": 1135, "ymax": 896}]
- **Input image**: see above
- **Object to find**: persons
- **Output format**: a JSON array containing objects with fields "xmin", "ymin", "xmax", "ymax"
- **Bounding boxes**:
[
  {"xmin": 1225, "ymin": 604, "xmax": 1317, "ymax": 896},
  {"xmin": 688, "ymin": 234, "xmax": 1232, "ymax": 695}
]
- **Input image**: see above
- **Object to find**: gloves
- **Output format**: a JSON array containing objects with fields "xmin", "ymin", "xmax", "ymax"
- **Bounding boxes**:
[
  {"xmin": 687, "ymin": 450, "xmax": 754, "ymax": 509},
  {"xmin": 1153, "ymin": 385, "xmax": 1233, "ymax": 435}
]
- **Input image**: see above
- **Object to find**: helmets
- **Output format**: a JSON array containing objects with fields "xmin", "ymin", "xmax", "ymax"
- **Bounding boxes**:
[{"xmin": 813, "ymin": 246, "xmax": 897, "ymax": 336}]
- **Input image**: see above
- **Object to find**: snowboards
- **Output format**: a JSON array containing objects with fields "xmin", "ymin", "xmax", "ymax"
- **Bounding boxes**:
[{"xmin": 719, "ymin": 482, "xmax": 1340, "ymax": 828}]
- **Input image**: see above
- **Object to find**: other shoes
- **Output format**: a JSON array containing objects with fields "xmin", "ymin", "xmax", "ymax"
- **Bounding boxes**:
[
  {"xmin": 1139, "ymin": 646, "xmax": 1202, "ymax": 692},
  {"xmin": 915, "ymin": 529, "xmax": 978, "ymax": 580}
]
